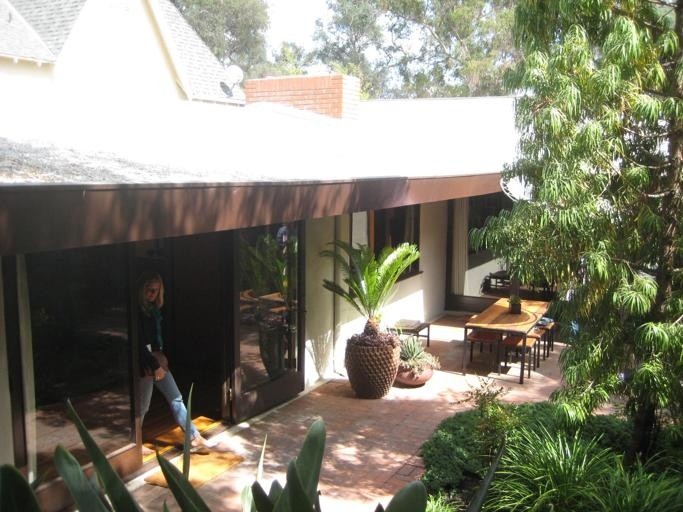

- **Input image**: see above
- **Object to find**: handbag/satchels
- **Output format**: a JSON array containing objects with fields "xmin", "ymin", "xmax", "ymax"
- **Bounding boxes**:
[{"xmin": 144, "ymin": 351, "xmax": 168, "ymax": 382}]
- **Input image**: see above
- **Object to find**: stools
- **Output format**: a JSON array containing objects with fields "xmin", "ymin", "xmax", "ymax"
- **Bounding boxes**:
[
  {"xmin": 500, "ymin": 322, "xmax": 555, "ymax": 378},
  {"xmin": 467, "ymin": 330, "xmax": 497, "ymax": 363}
]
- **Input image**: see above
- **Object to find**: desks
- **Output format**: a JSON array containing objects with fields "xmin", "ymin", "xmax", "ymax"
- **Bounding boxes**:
[
  {"xmin": 464, "ymin": 298, "xmax": 549, "ymax": 382},
  {"xmin": 386, "ymin": 321, "xmax": 430, "ymax": 346}
]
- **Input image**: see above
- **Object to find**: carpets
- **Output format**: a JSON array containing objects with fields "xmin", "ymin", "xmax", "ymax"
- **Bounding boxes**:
[{"xmin": 143, "ymin": 440, "xmax": 247, "ymax": 493}]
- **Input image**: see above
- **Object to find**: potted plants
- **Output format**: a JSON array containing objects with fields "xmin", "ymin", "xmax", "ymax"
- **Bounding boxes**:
[
  {"xmin": 508, "ymin": 294, "xmax": 521, "ymax": 314},
  {"xmin": 396, "ymin": 338, "xmax": 441, "ymax": 387},
  {"xmin": 241, "ymin": 230, "xmax": 291, "ymax": 377},
  {"xmin": 316, "ymin": 231, "xmax": 425, "ymax": 401}
]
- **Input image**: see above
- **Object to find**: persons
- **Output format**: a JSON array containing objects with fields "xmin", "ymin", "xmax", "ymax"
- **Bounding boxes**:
[{"xmin": 137, "ymin": 270, "xmax": 219, "ymax": 455}]
